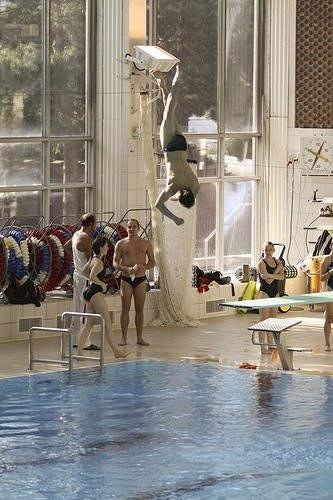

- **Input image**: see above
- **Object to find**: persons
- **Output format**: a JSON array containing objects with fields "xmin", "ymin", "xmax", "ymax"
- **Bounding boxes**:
[
  {"xmin": 75, "ymin": 239, "xmax": 131, "ymax": 361},
  {"xmin": 149, "ymin": 64, "xmax": 201, "ymax": 227},
  {"xmin": 258, "ymin": 242, "xmax": 284, "ymax": 354},
  {"xmin": 113, "ymin": 219, "xmax": 156, "ymax": 346},
  {"xmin": 72, "ymin": 213, "xmax": 101, "ymax": 351},
  {"xmin": 320, "ymin": 241, "xmax": 333, "ymax": 352}
]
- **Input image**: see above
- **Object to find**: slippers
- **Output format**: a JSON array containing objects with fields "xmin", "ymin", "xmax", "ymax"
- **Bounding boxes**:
[
  {"xmin": 73, "ymin": 345, "xmax": 78, "ymax": 347},
  {"xmin": 83, "ymin": 343, "xmax": 100, "ymax": 350}
]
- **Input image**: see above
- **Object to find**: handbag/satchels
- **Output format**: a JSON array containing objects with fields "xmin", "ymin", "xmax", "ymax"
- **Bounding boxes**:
[{"xmin": 4, "ymin": 277, "xmax": 40, "ymax": 307}]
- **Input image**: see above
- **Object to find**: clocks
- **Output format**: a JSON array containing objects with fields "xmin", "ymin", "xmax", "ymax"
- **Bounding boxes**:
[{"xmin": 303, "ymin": 139, "xmax": 333, "ymax": 170}]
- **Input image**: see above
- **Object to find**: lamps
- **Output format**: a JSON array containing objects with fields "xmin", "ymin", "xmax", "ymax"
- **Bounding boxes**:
[{"xmin": 126, "ymin": 46, "xmax": 180, "ymax": 87}]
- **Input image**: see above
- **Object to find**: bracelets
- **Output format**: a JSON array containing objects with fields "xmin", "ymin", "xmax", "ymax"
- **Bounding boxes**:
[{"xmin": 328, "ymin": 270, "xmax": 331, "ymax": 275}]
